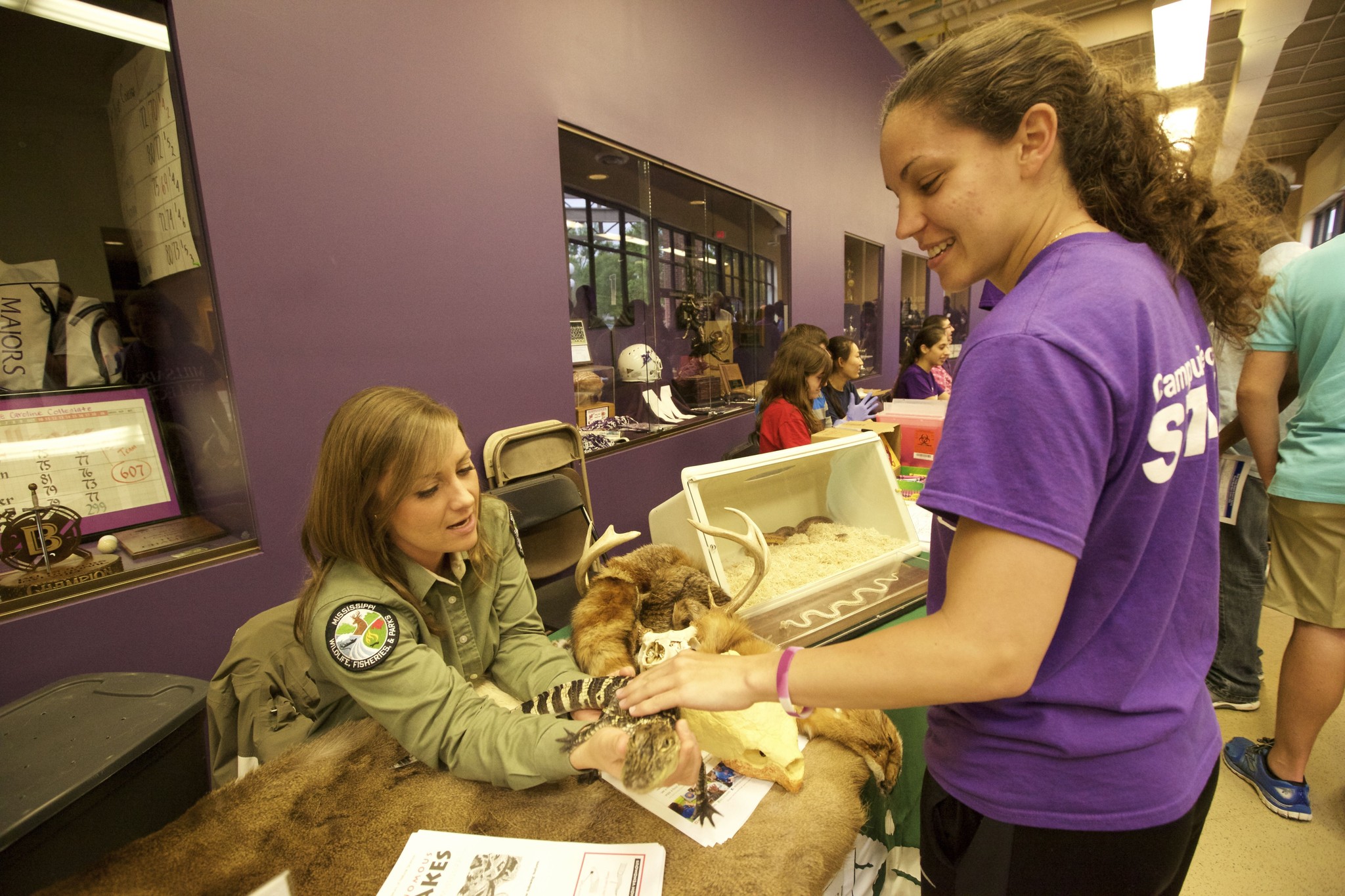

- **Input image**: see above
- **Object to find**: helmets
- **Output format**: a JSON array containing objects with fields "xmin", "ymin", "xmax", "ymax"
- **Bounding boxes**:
[{"xmin": 617, "ymin": 344, "xmax": 663, "ymax": 382}]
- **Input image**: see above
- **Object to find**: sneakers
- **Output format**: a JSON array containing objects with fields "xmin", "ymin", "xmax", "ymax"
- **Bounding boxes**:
[{"xmin": 1222, "ymin": 735, "xmax": 1313, "ymax": 823}]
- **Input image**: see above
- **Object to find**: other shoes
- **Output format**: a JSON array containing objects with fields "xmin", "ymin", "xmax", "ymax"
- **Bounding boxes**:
[{"xmin": 1203, "ymin": 681, "xmax": 1261, "ymax": 711}]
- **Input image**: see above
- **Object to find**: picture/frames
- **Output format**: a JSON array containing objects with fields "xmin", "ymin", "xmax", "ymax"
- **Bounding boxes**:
[
  {"xmin": 717, "ymin": 362, "xmax": 748, "ymax": 395},
  {"xmin": 569, "ymin": 318, "xmax": 594, "ymax": 366},
  {"xmin": 0, "ymin": 381, "xmax": 190, "ymax": 559},
  {"xmin": 111, "ymin": 512, "xmax": 228, "ymax": 559}
]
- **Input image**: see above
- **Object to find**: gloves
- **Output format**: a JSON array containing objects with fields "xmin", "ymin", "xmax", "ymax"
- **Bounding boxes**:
[
  {"xmin": 846, "ymin": 391, "xmax": 880, "ymax": 422},
  {"xmin": 833, "ymin": 419, "xmax": 846, "ymax": 427}
]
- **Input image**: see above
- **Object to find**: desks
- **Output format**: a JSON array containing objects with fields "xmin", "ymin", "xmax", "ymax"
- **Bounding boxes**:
[{"xmin": 29, "ymin": 465, "xmax": 929, "ymax": 896}]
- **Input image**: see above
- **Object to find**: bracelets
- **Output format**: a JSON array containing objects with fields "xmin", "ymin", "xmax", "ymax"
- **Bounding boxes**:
[{"xmin": 776, "ymin": 647, "xmax": 815, "ymax": 718}]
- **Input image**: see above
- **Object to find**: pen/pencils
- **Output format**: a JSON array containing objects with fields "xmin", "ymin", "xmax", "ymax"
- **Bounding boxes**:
[{"xmin": 831, "ymin": 426, "xmax": 873, "ymax": 432}]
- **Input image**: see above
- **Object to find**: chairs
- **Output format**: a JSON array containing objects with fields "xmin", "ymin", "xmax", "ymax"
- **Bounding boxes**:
[
  {"xmin": 205, "ymin": 600, "xmax": 370, "ymax": 792},
  {"xmin": 486, "ymin": 472, "xmax": 611, "ymax": 632},
  {"xmin": 482, "ymin": 419, "xmax": 601, "ymax": 579}
]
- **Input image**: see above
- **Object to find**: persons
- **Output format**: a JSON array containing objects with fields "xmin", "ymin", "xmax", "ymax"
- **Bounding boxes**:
[
  {"xmin": 617, "ymin": 15, "xmax": 1270, "ymax": 896},
  {"xmin": 860, "ymin": 296, "xmax": 960, "ymax": 351},
  {"xmin": 1219, "ymin": 235, "xmax": 1345, "ymax": 823},
  {"xmin": 1207, "ymin": 167, "xmax": 1313, "ymax": 710},
  {"xmin": 750, "ymin": 313, "xmax": 954, "ymax": 455},
  {"xmin": 206, "ymin": 386, "xmax": 703, "ymax": 793},
  {"xmin": 571, "ymin": 285, "xmax": 786, "ymax": 348}
]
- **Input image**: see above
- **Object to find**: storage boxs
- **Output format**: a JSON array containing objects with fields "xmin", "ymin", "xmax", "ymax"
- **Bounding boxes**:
[
  {"xmin": 648, "ymin": 430, "xmax": 922, "ymax": 622},
  {"xmin": 811, "ymin": 426, "xmax": 864, "ymax": 443},
  {"xmin": 835, "ymin": 418, "xmax": 902, "ymax": 462}
]
